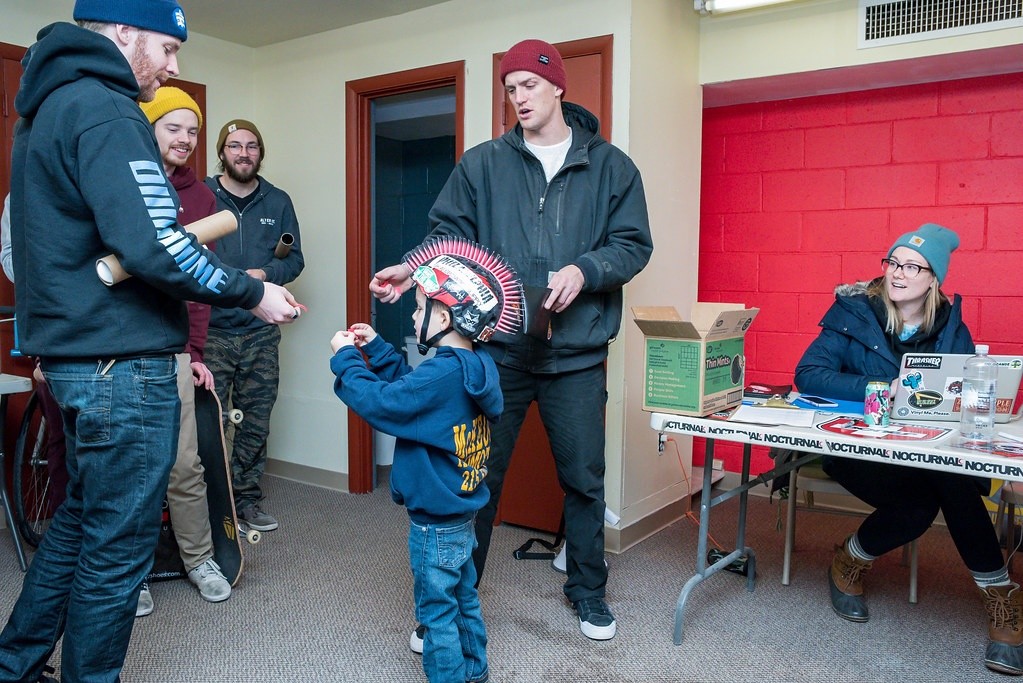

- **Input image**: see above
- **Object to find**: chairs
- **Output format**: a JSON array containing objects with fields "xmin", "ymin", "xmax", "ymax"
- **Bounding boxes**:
[
  {"xmin": 781, "ymin": 450, "xmax": 919, "ymax": 605},
  {"xmin": 993, "ymin": 403, "xmax": 1023, "ymax": 578}
]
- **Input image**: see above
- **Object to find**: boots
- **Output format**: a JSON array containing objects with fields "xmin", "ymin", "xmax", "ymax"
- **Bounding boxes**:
[
  {"xmin": 979, "ymin": 579, "xmax": 1023, "ymax": 675},
  {"xmin": 827, "ymin": 533, "xmax": 876, "ymax": 623}
]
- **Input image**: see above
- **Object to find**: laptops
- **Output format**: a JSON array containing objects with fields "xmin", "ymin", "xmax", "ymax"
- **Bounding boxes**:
[{"xmin": 888, "ymin": 353, "xmax": 1023, "ymax": 424}]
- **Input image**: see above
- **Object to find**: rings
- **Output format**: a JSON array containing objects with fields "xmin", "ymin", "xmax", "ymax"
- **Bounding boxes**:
[{"xmin": 291, "ymin": 308, "xmax": 299, "ymax": 319}]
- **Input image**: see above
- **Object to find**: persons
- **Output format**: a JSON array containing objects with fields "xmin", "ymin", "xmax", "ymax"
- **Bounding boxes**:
[
  {"xmin": 369, "ymin": 37, "xmax": 657, "ymax": 654},
  {"xmin": 0, "ymin": 188, "xmax": 71, "ymax": 510},
  {"xmin": 0, "ymin": 0, "xmax": 307, "ymax": 683},
  {"xmin": 793, "ymin": 221, "xmax": 1022, "ymax": 679},
  {"xmin": 202, "ymin": 118, "xmax": 307, "ymax": 533},
  {"xmin": 330, "ymin": 236, "xmax": 526, "ymax": 682},
  {"xmin": 123, "ymin": 85, "xmax": 233, "ymax": 618}
]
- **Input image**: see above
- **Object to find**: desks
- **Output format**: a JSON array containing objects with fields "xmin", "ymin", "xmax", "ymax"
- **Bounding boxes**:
[
  {"xmin": 1, "ymin": 372, "xmax": 35, "ymax": 572},
  {"xmin": 647, "ymin": 386, "xmax": 1023, "ymax": 649}
]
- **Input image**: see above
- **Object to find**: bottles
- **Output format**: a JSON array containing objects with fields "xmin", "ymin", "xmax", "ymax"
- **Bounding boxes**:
[{"xmin": 960, "ymin": 344, "xmax": 998, "ymax": 440}]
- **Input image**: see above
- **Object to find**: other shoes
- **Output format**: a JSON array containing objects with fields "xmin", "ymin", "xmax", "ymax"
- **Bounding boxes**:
[{"xmin": 32, "ymin": 674, "xmax": 59, "ymax": 683}]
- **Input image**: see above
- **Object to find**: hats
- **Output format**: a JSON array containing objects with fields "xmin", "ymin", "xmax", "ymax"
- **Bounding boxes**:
[
  {"xmin": 498, "ymin": 39, "xmax": 567, "ymax": 102},
  {"xmin": 72, "ymin": 0, "xmax": 187, "ymax": 43},
  {"xmin": 138, "ymin": 86, "xmax": 203, "ymax": 135},
  {"xmin": 216, "ymin": 119, "xmax": 265, "ymax": 162},
  {"xmin": 885, "ymin": 223, "xmax": 960, "ymax": 289}
]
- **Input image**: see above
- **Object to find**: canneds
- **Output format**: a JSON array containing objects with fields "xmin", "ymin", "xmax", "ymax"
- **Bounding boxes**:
[{"xmin": 863, "ymin": 381, "xmax": 890, "ymax": 427}]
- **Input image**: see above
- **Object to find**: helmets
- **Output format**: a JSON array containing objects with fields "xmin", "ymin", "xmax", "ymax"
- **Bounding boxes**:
[{"xmin": 410, "ymin": 253, "xmax": 504, "ymax": 345}]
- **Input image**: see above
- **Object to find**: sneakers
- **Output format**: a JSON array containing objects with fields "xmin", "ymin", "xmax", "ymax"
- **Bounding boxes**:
[
  {"xmin": 235, "ymin": 496, "xmax": 279, "ymax": 532},
  {"xmin": 135, "ymin": 578, "xmax": 154, "ymax": 618},
  {"xmin": 187, "ymin": 558, "xmax": 232, "ymax": 603},
  {"xmin": 572, "ymin": 597, "xmax": 617, "ymax": 640},
  {"xmin": 409, "ymin": 624, "xmax": 425, "ymax": 654}
]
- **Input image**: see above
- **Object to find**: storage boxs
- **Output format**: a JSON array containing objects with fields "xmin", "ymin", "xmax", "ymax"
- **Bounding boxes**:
[{"xmin": 631, "ymin": 302, "xmax": 760, "ymax": 418}]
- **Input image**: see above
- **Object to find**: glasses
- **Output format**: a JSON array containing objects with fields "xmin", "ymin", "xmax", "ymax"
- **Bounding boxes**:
[
  {"xmin": 880, "ymin": 258, "xmax": 933, "ymax": 279},
  {"xmin": 224, "ymin": 144, "xmax": 262, "ymax": 156}
]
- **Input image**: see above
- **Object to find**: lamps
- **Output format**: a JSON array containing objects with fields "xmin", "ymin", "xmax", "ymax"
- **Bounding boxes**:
[{"xmin": 691, "ymin": 0, "xmax": 800, "ymax": 20}]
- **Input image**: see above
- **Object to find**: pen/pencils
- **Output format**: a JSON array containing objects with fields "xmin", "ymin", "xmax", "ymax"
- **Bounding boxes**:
[
  {"xmin": 786, "ymin": 405, "xmax": 800, "ymax": 409},
  {"xmin": 740, "ymin": 400, "xmax": 762, "ymax": 405}
]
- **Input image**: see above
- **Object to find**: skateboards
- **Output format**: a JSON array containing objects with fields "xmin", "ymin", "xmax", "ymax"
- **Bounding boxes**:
[{"xmin": 194, "ymin": 378, "xmax": 261, "ymax": 588}]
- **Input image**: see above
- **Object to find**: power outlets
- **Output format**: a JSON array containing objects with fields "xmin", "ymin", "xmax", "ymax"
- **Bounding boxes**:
[
  {"xmin": 712, "ymin": 459, "xmax": 724, "ymax": 471},
  {"xmin": 658, "ymin": 434, "xmax": 665, "ymax": 453}
]
